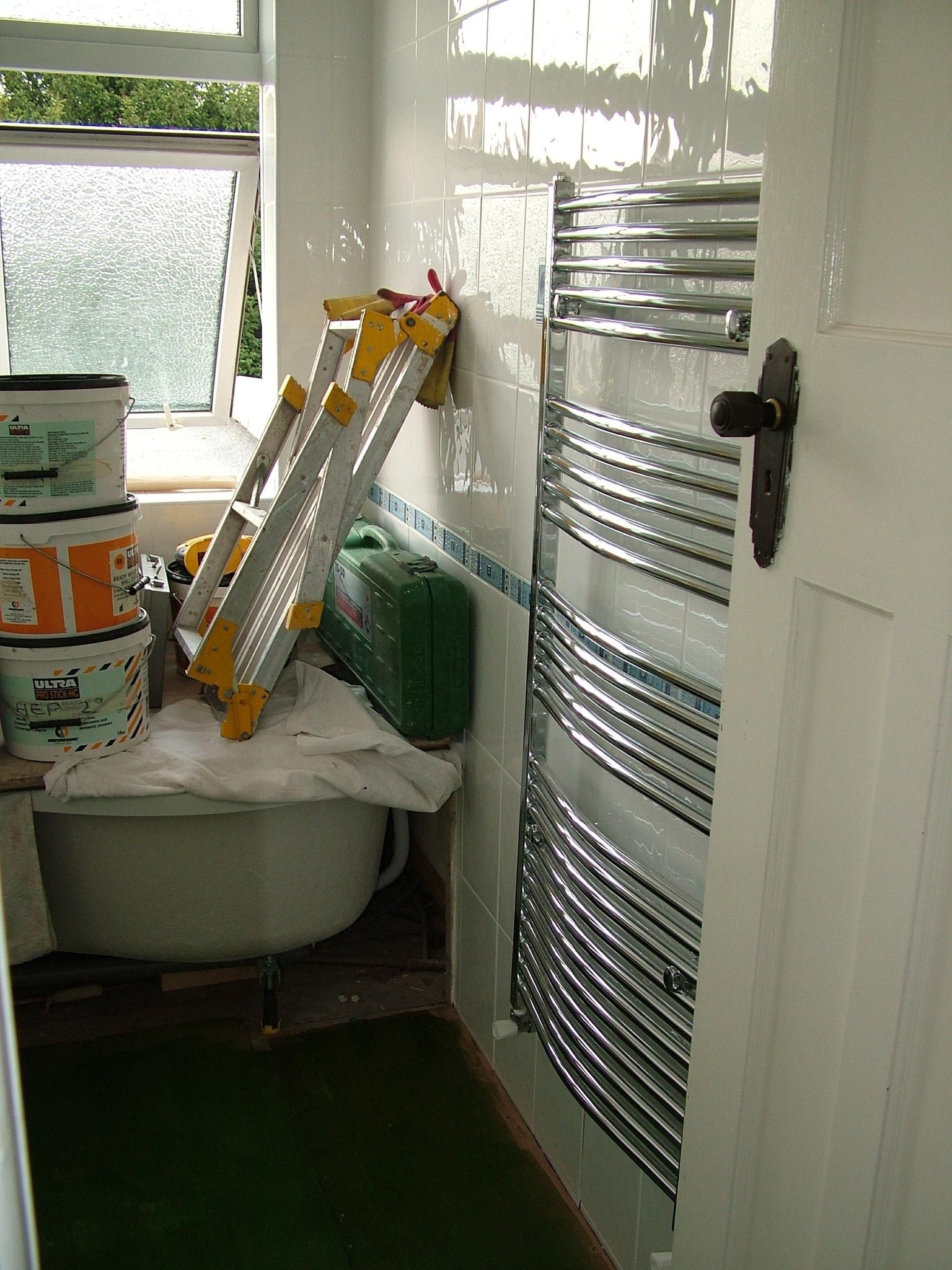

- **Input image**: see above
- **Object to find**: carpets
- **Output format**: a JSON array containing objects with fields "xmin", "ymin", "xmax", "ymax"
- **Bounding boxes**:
[{"xmin": 16, "ymin": 1013, "xmax": 593, "ymax": 1270}]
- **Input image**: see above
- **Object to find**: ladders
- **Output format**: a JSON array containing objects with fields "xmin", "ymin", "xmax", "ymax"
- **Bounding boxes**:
[{"xmin": 169, "ymin": 291, "xmax": 459, "ymax": 739}]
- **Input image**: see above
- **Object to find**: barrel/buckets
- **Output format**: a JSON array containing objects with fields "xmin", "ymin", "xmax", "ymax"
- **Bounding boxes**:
[
  {"xmin": 0, "ymin": 491, "xmax": 151, "ymax": 640},
  {"xmin": 0, "ymin": 373, "xmax": 136, "ymax": 515},
  {"xmin": 165, "ymin": 559, "xmax": 236, "ymax": 678},
  {"xmin": 0, "ymin": 607, "xmax": 156, "ymax": 762}
]
